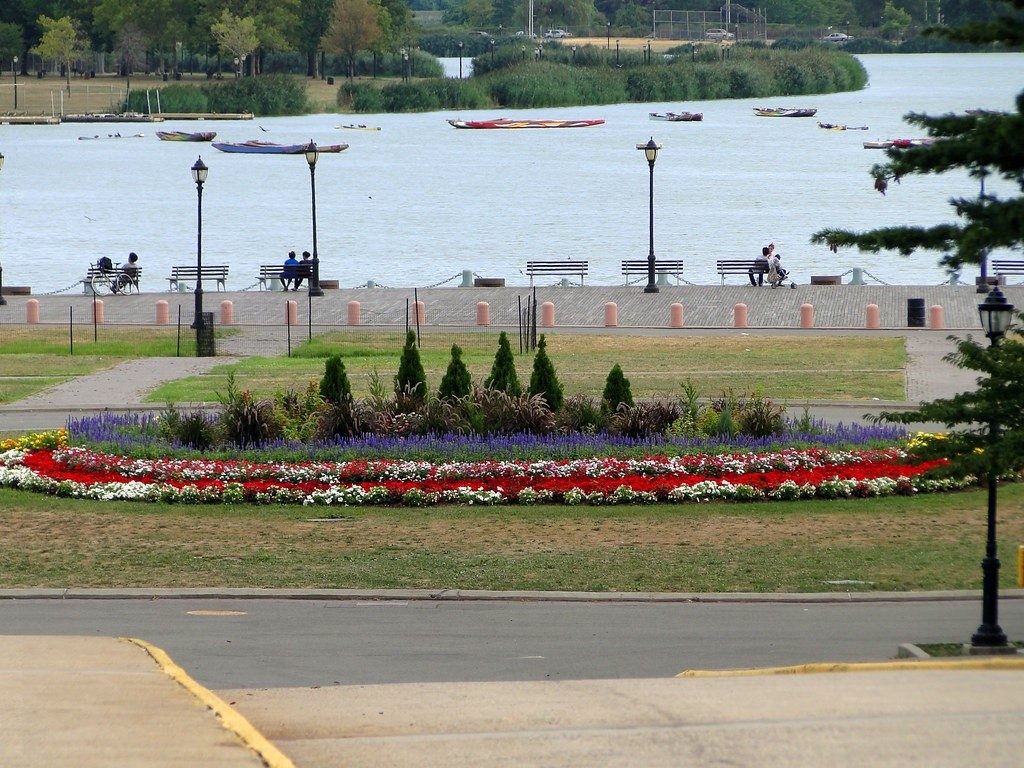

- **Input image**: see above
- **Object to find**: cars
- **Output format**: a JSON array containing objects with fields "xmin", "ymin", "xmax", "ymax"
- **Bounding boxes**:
[
  {"xmin": 468, "ymin": 31, "xmax": 493, "ymax": 39},
  {"xmin": 515, "ymin": 29, "xmax": 573, "ymax": 38},
  {"xmin": 824, "ymin": 33, "xmax": 856, "ymax": 42}
]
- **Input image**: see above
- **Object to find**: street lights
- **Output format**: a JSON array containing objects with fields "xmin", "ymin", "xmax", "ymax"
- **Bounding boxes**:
[
  {"xmin": 572, "ymin": 39, "xmax": 730, "ymax": 66},
  {"xmin": 643, "ymin": 136, "xmax": 660, "ymax": 294},
  {"xmin": 734, "ymin": 22, "xmax": 739, "ymax": 40},
  {"xmin": 13, "ymin": 55, "xmax": 18, "ymax": 109},
  {"xmin": 606, "ymin": 21, "xmax": 611, "ymax": 50},
  {"xmin": 969, "ymin": 281, "xmax": 1015, "ymax": 645},
  {"xmin": 190, "ymin": 154, "xmax": 208, "ymax": 330},
  {"xmin": 304, "ymin": 139, "xmax": 325, "ymax": 297},
  {"xmin": 234, "ymin": 53, "xmax": 247, "ymax": 81},
  {"xmin": 846, "ymin": 19, "xmax": 850, "ymax": 41},
  {"xmin": 400, "ymin": 38, "xmax": 544, "ymax": 85}
]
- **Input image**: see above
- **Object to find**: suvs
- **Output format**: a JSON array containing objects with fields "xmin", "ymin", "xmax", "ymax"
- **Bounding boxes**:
[{"xmin": 705, "ymin": 29, "xmax": 734, "ymax": 40}]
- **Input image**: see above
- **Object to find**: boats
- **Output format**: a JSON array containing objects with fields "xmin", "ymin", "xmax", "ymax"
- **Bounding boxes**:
[
  {"xmin": 649, "ymin": 111, "xmax": 704, "ymax": 121},
  {"xmin": 863, "ymin": 138, "xmax": 934, "ymax": 150},
  {"xmin": 753, "ymin": 106, "xmax": 817, "ymax": 117},
  {"xmin": 211, "ymin": 139, "xmax": 350, "ymax": 154},
  {"xmin": 155, "ymin": 130, "xmax": 217, "ymax": 141},
  {"xmin": 816, "ymin": 120, "xmax": 869, "ymax": 130},
  {"xmin": 445, "ymin": 117, "xmax": 606, "ymax": 128},
  {"xmin": 334, "ymin": 125, "xmax": 382, "ymax": 130},
  {"xmin": 78, "ymin": 132, "xmax": 145, "ymax": 140}
]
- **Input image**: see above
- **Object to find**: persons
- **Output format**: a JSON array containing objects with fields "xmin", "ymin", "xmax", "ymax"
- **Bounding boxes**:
[
  {"xmin": 110, "ymin": 252, "xmax": 138, "ymax": 294},
  {"xmin": 749, "ymin": 243, "xmax": 790, "ymax": 287},
  {"xmin": 279, "ymin": 251, "xmax": 299, "ymax": 291},
  {"xmin": 292, "ymin": 251, "xmax": 313, "ymax": 291}
]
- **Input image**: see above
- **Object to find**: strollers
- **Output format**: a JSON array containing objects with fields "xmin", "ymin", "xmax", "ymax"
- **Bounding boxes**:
[{"xmin": 768, "ymin": 254, "xmax": 797, "ymax": 290}]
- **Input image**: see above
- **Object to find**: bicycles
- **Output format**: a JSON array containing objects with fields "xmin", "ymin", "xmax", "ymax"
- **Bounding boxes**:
[{"xmin": 90, "ymin": 262, "xmax": 134, "ymax": 296}]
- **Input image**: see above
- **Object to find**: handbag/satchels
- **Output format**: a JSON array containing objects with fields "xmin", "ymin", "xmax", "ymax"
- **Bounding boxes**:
[{"xmin": 767, "ymin": 265, "xmax": 778, "ymax": 283}]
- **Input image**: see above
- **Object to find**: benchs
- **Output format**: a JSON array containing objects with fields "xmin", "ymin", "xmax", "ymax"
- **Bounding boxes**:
[
  {"xmin": 256, "ymin": 264, "xmax": 313, "ymax": 291},
  {"xmin": 621, "ymin": 260, "xmax": 683, "ymax": 285},
  {"xmin": 526, "ymin": 261, "xmax": 588, "ymax": 286},
  {"xmin": 716, "ymin": 260, "xmax": 769, "ymax": 286},
  {"xmin": 79, "ymin": 267, "xmax": 141, "ymax": 294},
  {"xmin": 166, "ymin": 266, "xmax": 229, "ymax": 293},
  {"xmin": 992, "ymin": 260, "xmax": 1024, "ymax": 285}
]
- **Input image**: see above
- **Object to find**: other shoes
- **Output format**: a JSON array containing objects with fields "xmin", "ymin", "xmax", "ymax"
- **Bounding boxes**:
[
  {"xmin": 778, "ymin": 284, "xmax": 785, "ymax": 286},
  {"xmin": 754, "ymin": 283, "xmax": 757, "ymax": 286},
  {"xmin": 282, "ymin": 288, "xmax": 288, "ymax": 291},
  {"xmin": 291, "ymin": 288, "xmax": 297, "ymax": 291},
  {"xmin": 110, "ymin": 286, "xmax": 116, "ymax": 294},
  {"xmin": 759, "ymin": 284, "xmax": 763, "ymax": 286}
]
